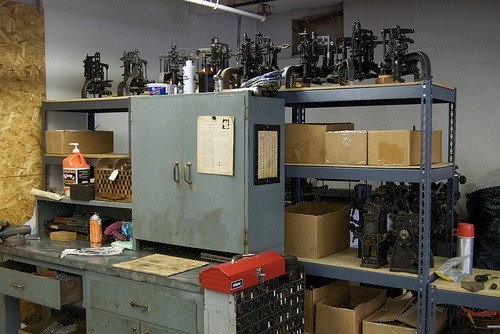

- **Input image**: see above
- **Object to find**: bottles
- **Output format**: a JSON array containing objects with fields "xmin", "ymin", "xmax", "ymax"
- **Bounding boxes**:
[
  {"xmin": 182, "ymin": 60, "xmax": 195, "ymax": 94},
  {"xmin": 90, "ymin": 212, "xmax": 102, "ymax": 246}
]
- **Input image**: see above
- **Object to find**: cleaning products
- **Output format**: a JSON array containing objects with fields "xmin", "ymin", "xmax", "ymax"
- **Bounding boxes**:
[
  {"xmin": 62, "ymin": 143, "xmax": 90, "ymax": 196},
  {"xmin": 182, "ymin": 60, "xmax": 195, "ymax": 94}
]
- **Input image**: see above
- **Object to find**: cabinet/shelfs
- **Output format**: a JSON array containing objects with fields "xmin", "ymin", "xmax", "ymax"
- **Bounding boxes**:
[
  {"xmin": 41, "ymin": 81, "xmax": 457, "ymax": 334},
  {"xmin": 428, "ymin": 267, "xmax": 500, "ymax": 334},
  {"xmin": 0, "ymin": 234, "xmax": 203, "ymax": 334}
]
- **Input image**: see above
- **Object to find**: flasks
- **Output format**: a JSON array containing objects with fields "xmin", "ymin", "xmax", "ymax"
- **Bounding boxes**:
[{"xmin": 452, "ymin": 223, "xmax": 475, "ymax": 275}]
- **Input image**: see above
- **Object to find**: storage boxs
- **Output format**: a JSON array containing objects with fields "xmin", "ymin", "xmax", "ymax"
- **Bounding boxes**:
[
  {"xmin": 305, "ymin": 279, "xmax": 448, "ymax": 334},
  {"xmin": 283, "ymin": 201, "xmax": 349, "ymax": 259},
  {"xmin": 45, "ymin": 129, "xmax": 113, "ymax": 154},
  {"xmin": 283, "ymin": 122, "xmax": 442, "ymax": 167}
]
